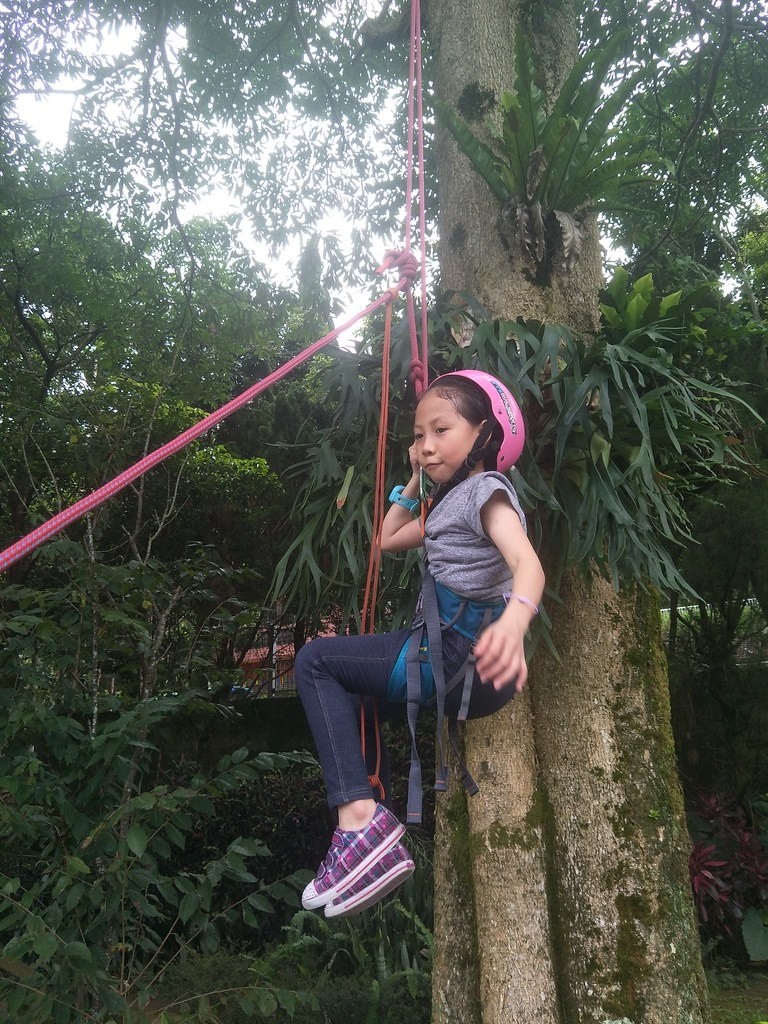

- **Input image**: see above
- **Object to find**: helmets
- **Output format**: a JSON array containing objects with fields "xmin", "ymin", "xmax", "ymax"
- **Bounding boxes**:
[{"xmin": 429, "ymin": 370, "xmax": 525, "ymax": 474}]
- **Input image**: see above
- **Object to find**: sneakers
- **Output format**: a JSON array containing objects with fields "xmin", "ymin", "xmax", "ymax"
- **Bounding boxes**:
[
  {"xmin": 301, "ymin": 802, "xmax": 406, "ymax": 911},
  {"xmin": 324, "ymin": 841, "xmax": 415, "ymax": 918}
]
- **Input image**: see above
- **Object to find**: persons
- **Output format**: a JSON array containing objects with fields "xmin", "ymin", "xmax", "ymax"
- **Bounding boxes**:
[{"xmin": 295, "ymin": 367, "xmax": 544, "ymax": 919}]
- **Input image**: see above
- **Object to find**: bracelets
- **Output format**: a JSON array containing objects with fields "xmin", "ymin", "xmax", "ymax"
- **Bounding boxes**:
[{"xmin": 503, "ymin": 592, "xmax": 540, "ymax": 617}]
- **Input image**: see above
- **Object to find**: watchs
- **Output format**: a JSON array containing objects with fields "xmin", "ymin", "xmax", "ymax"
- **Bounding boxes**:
[{"xmin": 389, "ymin": 485, "xmax": 419, "ymax": 513}]
